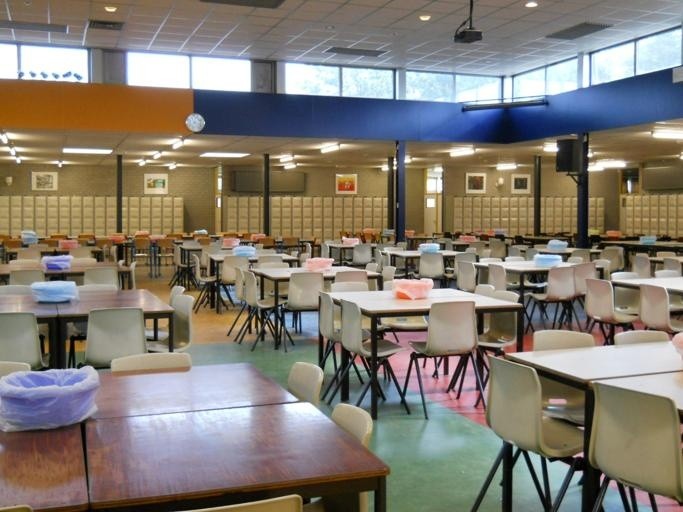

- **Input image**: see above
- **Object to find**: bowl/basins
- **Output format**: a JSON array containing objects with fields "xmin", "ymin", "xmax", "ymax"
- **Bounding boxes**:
[
  {"xmin": 304, "ymin": 257, "xmax": 335, "ymax": 272},
  {"xmin": 19, "ymin": 228, "xmax": 37, "ymax": 244},
  {"xmin": 110, "ymin": 226, "xmax": 267, "ymax": 258},
  {"xmin": 531, "ymin": 227, "xmax": 657, "ymax": 266},
  {"xmin": 31, "ymin": 279, "xmax": 77, "ymax": 304},
  {"xmin": 57, "ymin": 238, "xmax": 78, "ymax": 249},
  {"xmin": 41, "ymin": 254, "xmax": 74, "ymax": 270},
  {"xmin": 0, "ymin": 364, "xmax": 104, "ymax": 431},
  {"xmin": 340, "ymin": 226, "xmax": 510, "ymax": 255},
  {"xmin": 391, "ymin": 278, "xmax": 434, "ymax": 301}
]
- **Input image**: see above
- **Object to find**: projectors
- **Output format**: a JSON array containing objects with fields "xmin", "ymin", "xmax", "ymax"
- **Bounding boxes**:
[{"xmin": 454, "ymin": 28, "xmax": 482, "ymax": 44}]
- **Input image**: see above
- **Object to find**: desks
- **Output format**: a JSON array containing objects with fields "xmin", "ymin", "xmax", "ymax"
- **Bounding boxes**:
[{"xmin": 1, "ymin": 361, "xmax": 391, "ymax": 512}]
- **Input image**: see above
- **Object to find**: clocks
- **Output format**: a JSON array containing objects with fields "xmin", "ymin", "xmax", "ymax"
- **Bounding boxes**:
[{"xmin": 186, "ymin": 112, "xmax": 205, "ymax": 132}]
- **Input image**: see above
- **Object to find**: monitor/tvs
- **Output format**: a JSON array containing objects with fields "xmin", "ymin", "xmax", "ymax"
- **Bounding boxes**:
[{"xmin": 555, "ymin": 139, "xmax": 589, "ymax": 172}]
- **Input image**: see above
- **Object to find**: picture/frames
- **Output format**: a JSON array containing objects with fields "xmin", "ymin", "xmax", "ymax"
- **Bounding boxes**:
[
  {"xmin": 30, "ymin": 171, "xmax": 57, "ymax": 191},
  {"xmin": 510, "ymin": 173, "xmax": 531, "ymax": 195},
  {"xmin": 143, "ymin": 173, "xmax": 169, "ymax": 195},
  {"xmin": 335, "ymin": 173, "xmax": 357, "ymax": 195},
  {"xmin": 465, "ymin": 172, "xmax": 486, "ymax": 194}
]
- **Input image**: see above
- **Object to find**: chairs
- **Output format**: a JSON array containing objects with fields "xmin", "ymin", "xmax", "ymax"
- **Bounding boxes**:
[
  {"xmin": 587, "ymin": 382, "xmax": 682, "ymax": 511},
  {"xmin": 109, "ymin": 353, "xmax": 192, "ymax": 372},
  {"xmin": 473, "ymin": 356, "xmax": 587, "ymax": 512}
]
